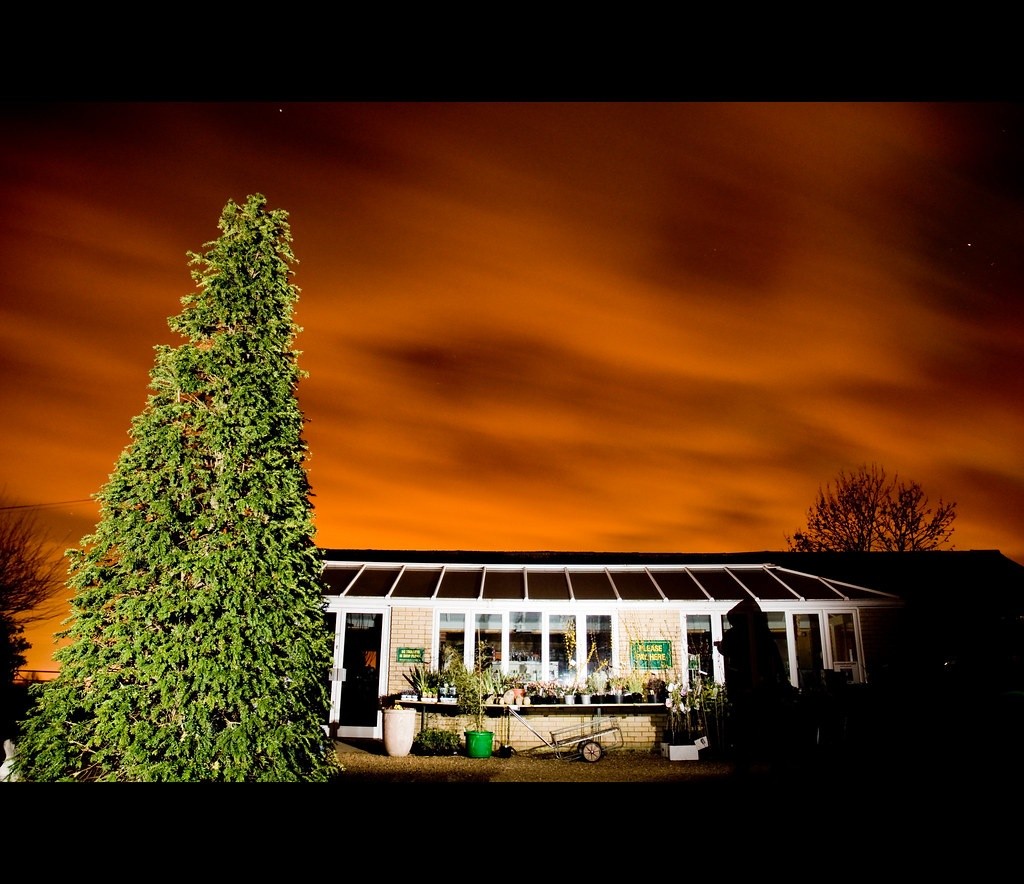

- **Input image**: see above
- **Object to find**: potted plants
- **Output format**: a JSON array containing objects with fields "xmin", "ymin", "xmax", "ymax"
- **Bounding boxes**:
[
  {"xmin": 464, "ymin": 628, "xmax": 496, "ymax": 758},
  {"xmin": 401, "ymin": 663, "xmax": 510, "ymax": 704},
  {"xmin": 495, "ymin": 711, "xmax": 512, "ymax": 758}
]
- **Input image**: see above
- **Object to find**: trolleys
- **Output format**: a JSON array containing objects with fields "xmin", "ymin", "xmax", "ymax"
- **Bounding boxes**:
[{"xmin": 503, "ymin": 706, "xmax": 624, "ymax": 764}]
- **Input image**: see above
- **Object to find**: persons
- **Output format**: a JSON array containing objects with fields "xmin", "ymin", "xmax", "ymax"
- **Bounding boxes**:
[{"xmin": 718, "ymin": 599, "xmax": 787, "ymax": 714}]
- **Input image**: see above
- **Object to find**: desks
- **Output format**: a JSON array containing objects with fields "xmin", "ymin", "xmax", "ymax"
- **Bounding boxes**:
[{"xmin": 395, "ymin": 699, "xmax": 665, "ymax": 746}]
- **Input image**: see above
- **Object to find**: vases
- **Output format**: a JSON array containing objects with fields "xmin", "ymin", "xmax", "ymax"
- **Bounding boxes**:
[
  {"xmin": 660, "ymin": 742, "xmax": 700, "ymax": 761},
  {"xmin": 648, "ymin": 693, "xmax": 657, "ymax": 702},
  {"xmin": 633, "ymin": 695, "xmax": 642, "ymax": 702},
  {"xmin": 516, "ymin": 698, "xmax": 523, "ymax": 704},
  {"xmin": 382, "ymin": 708, "xmax": 416, "ymax": 758},
  {"xmin": 574, "ymin": 693, "xmax": 582, "ymax": 704},
  {"xmin": 615, "ymin": 695, "xmax": 623, "ymax": 703},
  {"xmin": 513, "ymin": 689, "xmax": 525, "ymax": 703},
  {"xmin": 523, "ymin": 698, "xmax": 531, "ymax": 705},
  {"xmin": 564, "ymin": 695, "xmax": 575, "ymax": 704},
  {"xmin": 598, "ymin": 695, "xmax": 605, "ymax": 703},
  {"xmin": 580, "ymin": 695, "xmax": 591, "ymax": 704}
]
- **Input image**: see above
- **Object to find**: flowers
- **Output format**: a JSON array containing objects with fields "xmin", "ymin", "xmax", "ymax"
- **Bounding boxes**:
[
  {"xmin": 666, "ymin": 615, "xmax": 728, "ymax": 745},
  {"xmin": 507, "ymin": 613, "xmax": 666, "ymax": 698},
  {"xmin": 387, "ymin": 704, "xmax": 412, "ymax": 710}
]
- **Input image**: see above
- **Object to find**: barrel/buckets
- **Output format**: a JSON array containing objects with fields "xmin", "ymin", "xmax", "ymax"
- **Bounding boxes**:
[{"xmin": 382, "ymin": 709, "xmax": 417, "ymax": 757}]
